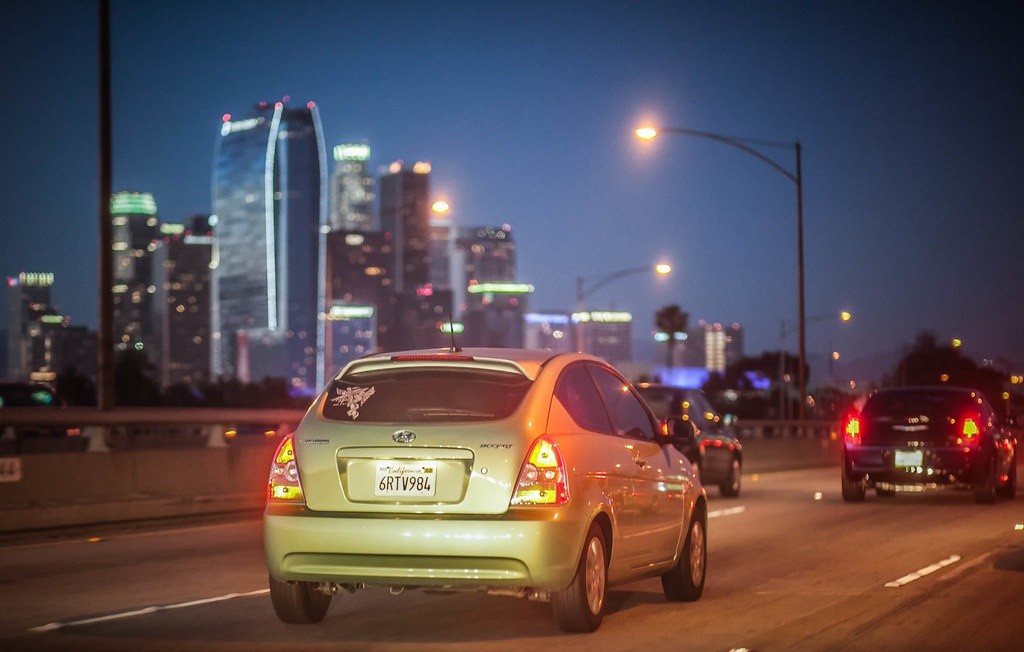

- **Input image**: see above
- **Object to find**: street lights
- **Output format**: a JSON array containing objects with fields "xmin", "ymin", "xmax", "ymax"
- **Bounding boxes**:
[
  {"xmin": 779, "ymin": 312, "xmax": 851, "ymax": 421},
  {"xmin": 635, "ymin": 125, "xmax": 807, "ymax": 420},
  {"xmin": 575, "ymin": 263, "xmax": 671, "ymax": 352}
]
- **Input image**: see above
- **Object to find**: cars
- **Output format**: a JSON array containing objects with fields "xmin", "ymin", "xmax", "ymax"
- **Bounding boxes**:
[
  {"xmin": 612, "ymin": 383, "xmax": 743, "ymax": 498},
  {"xmin": 223, "ymin": 395, "xmax": 314, "ymax": 444},
  {"xmin": 263, "ymin": 346, "xmax": 709, "ymax": 633},
  {"xmin": 995, "ymin": 393, "xmax": 1024, "ymax": 427},
  {"xmin": 842, "ymin": 387, "xmax": 1018, "ymax": 500},
  {"xmin": 0, "ymin": 381, "xmax": 84, "ymax": 453},
  {"xmin": 761, "ymin": 387, "xmax": 855, "ymax": 434}
]
